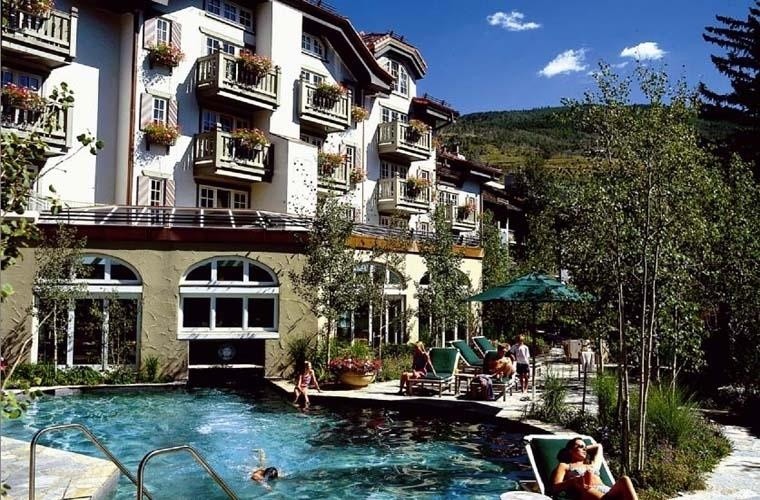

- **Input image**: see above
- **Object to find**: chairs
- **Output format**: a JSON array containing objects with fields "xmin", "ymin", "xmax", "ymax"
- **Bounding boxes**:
[
  {"xmin": 409, "ymin": 335, "xmax": 542, "ymax": 401},
  {"xmin": 523, "ymin": 434, "xmax": 616, "ymax": 499}
]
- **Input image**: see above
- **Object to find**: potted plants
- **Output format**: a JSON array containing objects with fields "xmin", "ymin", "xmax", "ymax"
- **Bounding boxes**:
[{"xmin": 329, "ymin": 358, "xmax": 381, "ymax": 386}]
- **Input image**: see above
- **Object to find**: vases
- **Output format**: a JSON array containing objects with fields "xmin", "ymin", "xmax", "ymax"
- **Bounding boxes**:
[{"xmin": 242, "ymin": 141, "xmax": 262, "ymax": 150}]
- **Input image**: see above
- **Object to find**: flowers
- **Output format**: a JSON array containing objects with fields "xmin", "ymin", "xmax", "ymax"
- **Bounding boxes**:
[
  {"xmin": 408, "ymin": 178, "xmax": 432, "ymax": 194},
  {"xmin": 143, "ymin": 119, "xmax": 181, "ymax": 146},
  {"xmin": 410, "ymin": 118, "xmax": 427, "ymax": 137},
  {"xmin": 242, "ymin": 49, "xmax": 273, "ymax": 78},
  {"xmin": 233, "ymin": 126, "xmax": 269, "ymax": 151},
  {"xmin": 150, "ymin": 42, "xmax": 184, "ymax": 68},
  {"xmin": 319, "ymin": 153, "xmax": 344, "ymax": 171},
  {"xmin": 349, "ymin": 103, "xmax": 368, "ymax": 122},
  {"xmin": 4, "ymin": 83, "xmax": 45, "ymax": 113},
  {"xmin": 317, "ymin": 82, "xmax": 345, "ymax": 102}
]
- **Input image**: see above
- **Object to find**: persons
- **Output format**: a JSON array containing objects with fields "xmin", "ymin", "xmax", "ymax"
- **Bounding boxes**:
[
  {"xmin": 553, "ymin": 437, "xmax": 639, "ymax": 500},
  {"xmin": 474, "ymin": 344, "xmax": 513, "ymax": 386},
  {"xmin": 398, "ymin": 341, "xmax": 442, "ymax": 395},
  {"xmin": 509, "ymin": 335, "xmax": 531, "ymax": 394},
  {"xmin": 251, "ymin": 467, "xmax": 278, "ymax": 487},
  {"xmin": 292, "ymin": 361, "xmax": 323, "ymax": 408}
]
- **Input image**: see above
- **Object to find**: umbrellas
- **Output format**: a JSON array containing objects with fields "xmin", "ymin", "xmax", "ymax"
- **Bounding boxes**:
[{"xmin": 460, "ymin": 271, "xmax": 585, "ymax": 402}]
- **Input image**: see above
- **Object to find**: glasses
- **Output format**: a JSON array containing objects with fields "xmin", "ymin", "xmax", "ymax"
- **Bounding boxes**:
[{"xmin": 575, "ymin": 444, "xmax": 585, "ymax": 448}]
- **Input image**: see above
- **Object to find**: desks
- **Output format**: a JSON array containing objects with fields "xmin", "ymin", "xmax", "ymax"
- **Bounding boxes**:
[{"xmin": 499, "ymin": 490, "xmax": 553, "ymax": 500}]
